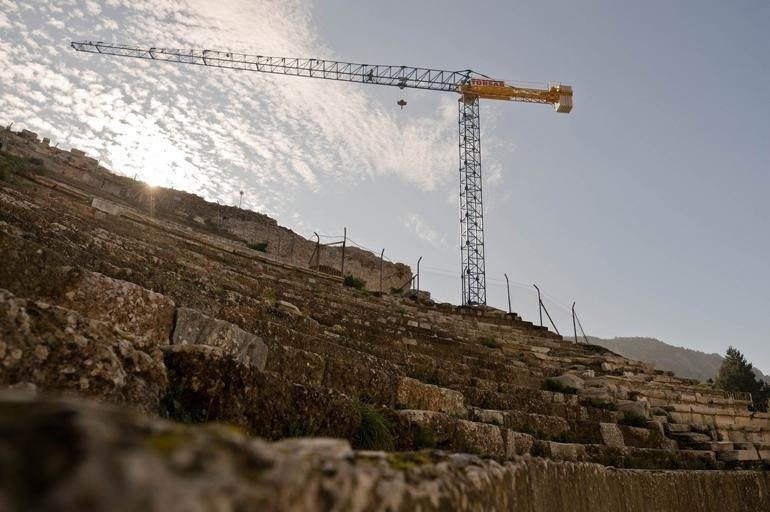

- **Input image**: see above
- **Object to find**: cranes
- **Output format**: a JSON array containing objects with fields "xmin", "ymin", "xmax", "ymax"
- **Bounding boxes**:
[{"xmin": 66, "ymin": 35, "xmax": 575, "ymax": 307}]
